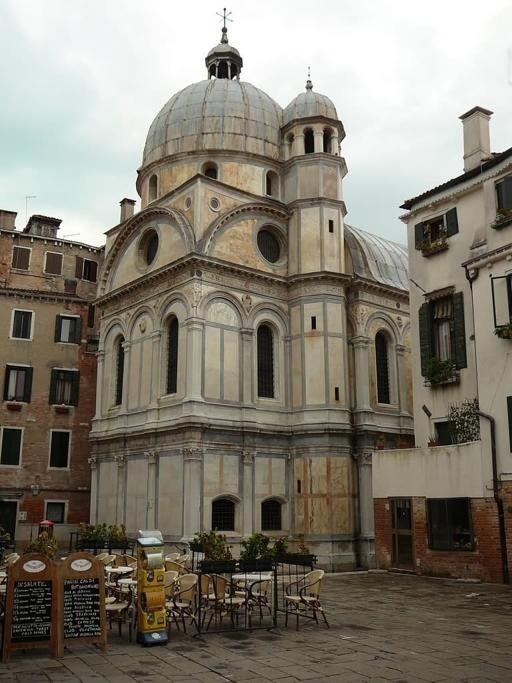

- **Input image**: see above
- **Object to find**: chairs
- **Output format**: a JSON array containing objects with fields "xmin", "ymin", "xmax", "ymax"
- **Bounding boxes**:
[{"xmin": 96, "ymin": 549, "xmax": 330, "ymax": 642}]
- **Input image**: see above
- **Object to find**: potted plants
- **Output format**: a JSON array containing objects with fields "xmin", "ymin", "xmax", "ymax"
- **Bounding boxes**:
[
  {"xmin": 490, "ymin": 208, "xmax": 512, "ymax": 229},
  {"xmin": 77, "ymin": 521, "xmax": 127, "ymax": 550},
  {"xmin": 423, "ymin": 357, "xmax": 458, "ymax": 387},
  {"xmin": 493, "ymin": 324, "xmax": 512, "ymax": 339},
  {"xmin": 188, "ymin": 528, "xmax": 315, "ymax": 572},
  {"xmin": 414, "ymin": 239, "xmax": 448, "ymax": 257}
]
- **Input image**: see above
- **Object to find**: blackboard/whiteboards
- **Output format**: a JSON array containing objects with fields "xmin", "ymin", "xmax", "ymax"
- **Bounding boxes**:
[{"xmin": 4, "ymin": 552, "xmax": 107, "ymax": 650}]
